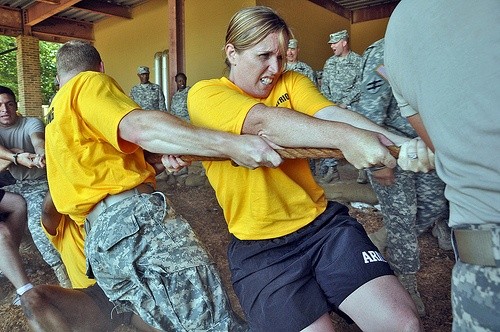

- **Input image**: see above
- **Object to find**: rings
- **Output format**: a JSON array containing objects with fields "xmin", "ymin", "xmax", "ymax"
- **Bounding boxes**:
[{"xmin": 407, "ymin": 152, "xmax": 418, "ymax": 160}]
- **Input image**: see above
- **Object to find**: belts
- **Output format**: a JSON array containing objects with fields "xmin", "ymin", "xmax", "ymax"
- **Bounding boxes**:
[
  {"xmin": 454, "ymin": 228, "xmax": 500, "ymax": 266},
  {"xmin": 84, "ymin": 184, "xmax": 156, "ymax": 234}
]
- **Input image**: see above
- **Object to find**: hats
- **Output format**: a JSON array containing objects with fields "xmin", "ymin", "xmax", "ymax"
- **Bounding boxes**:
[
  {"xmin": 328, "ymin": 30, "xmax": 349, "ymax": 44},
  {"xmin": 288, "ymin": 39, "xmax": 298, "ymax": 48},
  {"xmin": 138, "ymin": 66, "xmax": 150, "ymax": 74},
  {"xmin": 314, "ymin": 70, "xmax": 324, "ymax": 78}
]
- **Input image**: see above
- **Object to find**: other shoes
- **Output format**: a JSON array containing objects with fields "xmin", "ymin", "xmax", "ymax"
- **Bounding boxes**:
[
  {"xmin": 319, "ymin": 166, "xmax": 340, "ymax": 184},
  {"xmin": 398, "ymin": 272, "xmax": 426, "ymax": 317},
  {"xmin": 431, "ymin": 219, "xmax": 453, "ymax": 251},
  {"xmin": 357, "ymin": 170, "xmax": 368, "ymax": 184},
  {"xmin": 367, "ymin": 224, "xmax": 391, "ymax": 262}
]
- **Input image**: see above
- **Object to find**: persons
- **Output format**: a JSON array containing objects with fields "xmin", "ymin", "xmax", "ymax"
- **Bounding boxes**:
[{"xmin": 0, "ymin": 0, "xmax": 500, "ymax": 332}]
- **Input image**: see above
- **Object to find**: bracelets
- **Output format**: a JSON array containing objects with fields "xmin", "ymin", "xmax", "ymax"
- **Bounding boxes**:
[{"xmin": 13, "ymin": 153, "xmax": 20, "ymax": 166}]
